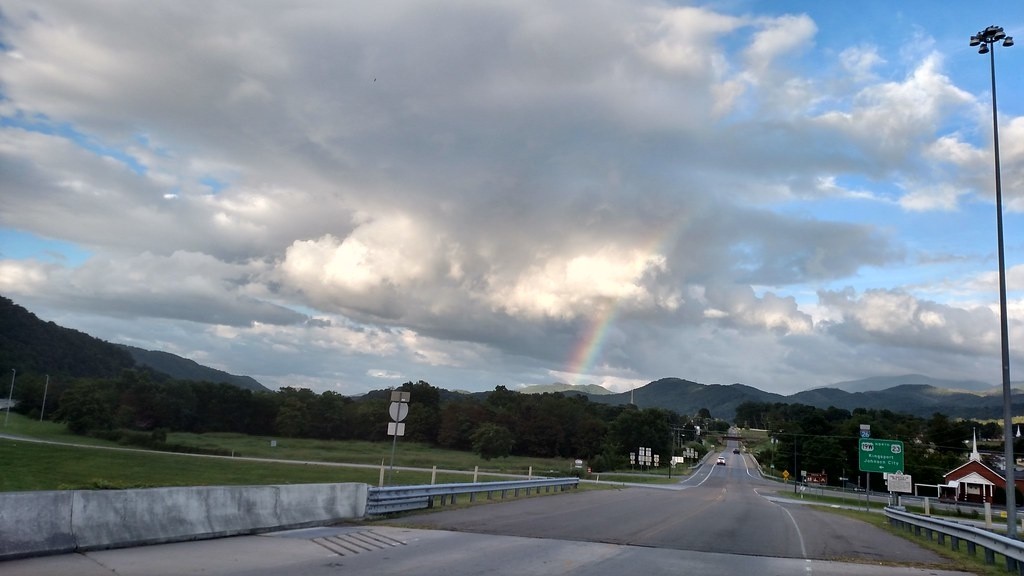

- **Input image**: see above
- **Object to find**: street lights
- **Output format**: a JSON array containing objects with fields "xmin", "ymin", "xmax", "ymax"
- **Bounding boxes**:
[
  {"xmin": 4, "ymin": 369, "xmax": 17, "ymax": 424},
  {"xmin": 40, "ymin": 374, "xmax": 50, "ymax": 422},
  {"xmin": 968, "ymin": 24, "xmax": 1018, "ymax": 540}
]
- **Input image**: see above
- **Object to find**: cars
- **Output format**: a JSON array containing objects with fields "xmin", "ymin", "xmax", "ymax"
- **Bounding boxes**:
[{"xmin": 717, "ymin": 457, "xmax": 727, "ymax": 465}]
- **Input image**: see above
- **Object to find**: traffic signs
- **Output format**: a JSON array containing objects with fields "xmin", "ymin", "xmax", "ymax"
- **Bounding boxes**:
[{"xmin": 858, "ymin": 438, "xmax": 905, "ymax": 476}]
